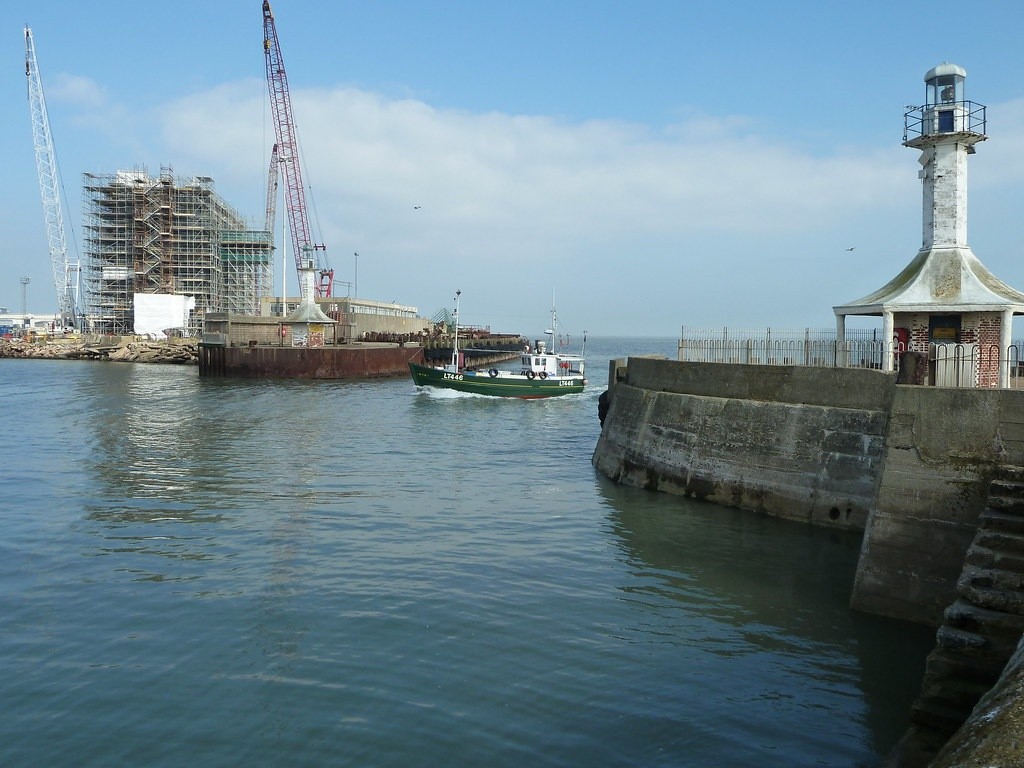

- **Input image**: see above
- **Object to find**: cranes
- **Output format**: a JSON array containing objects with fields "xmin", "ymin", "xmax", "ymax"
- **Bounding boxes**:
[
  {"xmin": 262, "ymin": 0, "xmax": 334, "ymax": 298},
  {"xmin": 24, "ymin": 23, "xmax": 82, "ymax": 334}
]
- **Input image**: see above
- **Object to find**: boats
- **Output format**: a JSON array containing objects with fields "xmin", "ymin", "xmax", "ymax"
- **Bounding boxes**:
[{"xmin": 408, "ymin": 287, "xmax": 587, "ymax": 399}]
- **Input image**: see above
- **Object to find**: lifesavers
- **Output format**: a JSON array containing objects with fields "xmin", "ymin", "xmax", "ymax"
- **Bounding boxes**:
[
  {"xmin": 527, "ymin": 371, "xmax": 535, "ymax": 380},
  {"xmin": 539, "ymin": 372, "xmax": 547, "ymax": 379},
  {"xmin": 489, "ymin": 369, "xmax": 498, "ymax": 378}
]
